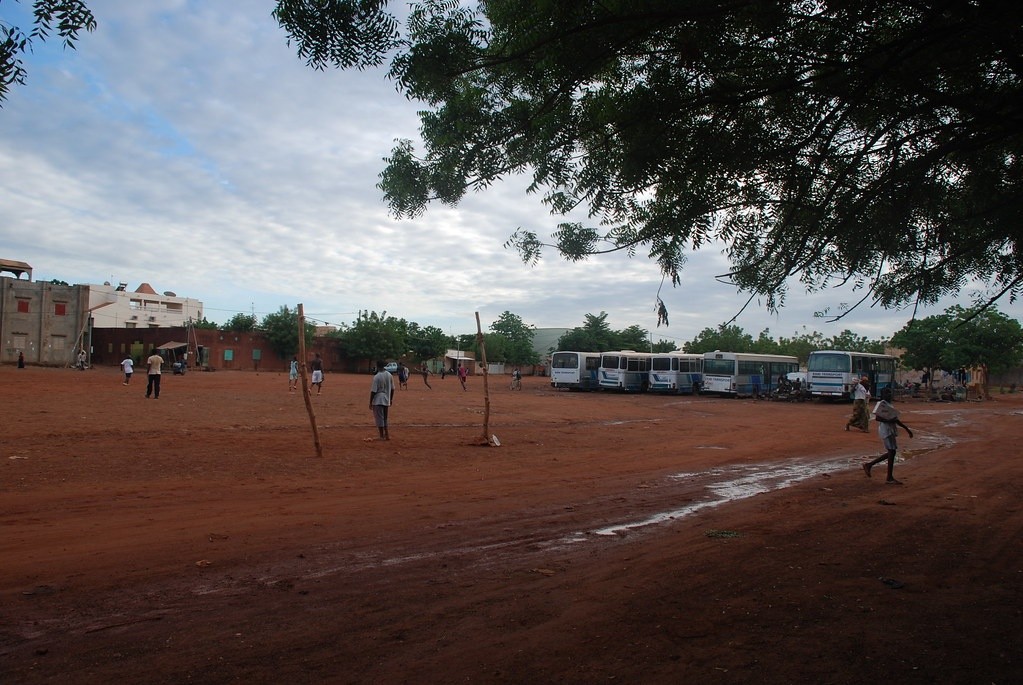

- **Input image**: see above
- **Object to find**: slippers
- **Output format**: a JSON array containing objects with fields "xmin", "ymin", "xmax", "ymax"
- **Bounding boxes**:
[
  {"xmin": 863, "ymin": 464, "xmax": 872, "ymax": 477},
  {"xmin": 885, "ymin": 479, "xmax": 903, "ymax": 485}
]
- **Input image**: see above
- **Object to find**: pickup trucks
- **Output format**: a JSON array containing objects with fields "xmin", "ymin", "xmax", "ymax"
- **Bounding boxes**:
[{"xmin": 384, "ymin": 362, "xmax": 398, "ymax": 373}]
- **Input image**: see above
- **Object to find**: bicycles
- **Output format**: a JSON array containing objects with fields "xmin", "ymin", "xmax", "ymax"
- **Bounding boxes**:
[{"xmin": 509, "ymin": 378, "xmax": 522, "ymax": 391}]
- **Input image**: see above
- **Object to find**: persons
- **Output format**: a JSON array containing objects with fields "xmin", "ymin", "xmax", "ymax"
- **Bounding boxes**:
[
  {"xmin": 456, "ymin": 364, "xmax": 467, "ymax": 392},
  {"xmin": 170, "ymin": 357, "xmax": 186, "ymax": 372},
  {"xmin": 18, "ymin": 351, "xmax": 26, "ymax": 369},
  {"xmin": 778, "ymin": 372, "xmax": 807, "ymax": 392},
  {"xmin": 306, "ymin": 353, "xmax": 324, "ymax": 396},
  {"xmin": 369, "ymin": 360, "xmax": 395, "ymax": 440},
  {"xmin": 289, "ymin": 355, "xmax": 303, "ymax": 391},
  {"xmin": 441, "ymin": 365, "xmax": 470, "ymax": 380},
  {"xmin": 511, "ymin": 367, "xmax": 522, "ymax": 388},
  {"xmin": 145, "ymin": 349, "xmax": 165, "ymax": 399},
  {"xmin": 894, "ymin": 379, "xmax": 923, "ymax": 395},
  {"xmin": 863, "ymin": 386, "xmax": 914, "ymax": 485},
  {"xmin": 77, "ymin": 349, "xmax": 89, "ymax": 370},
  {"xmin": 844, "ymin": 376, "xmax": 871, "ymax": 433},
  {"xmin": 119, "ymin": 352, "xmax": 141, "ymax": 386},
  {"xmin": 943, "ymin": 382, "xmax": 966, "ymax": 402},
  {"xmin": 397, "ymin": 363, "xmax": 410, "ymax": 390},
  {"xmin": 195, "ymin": 353, "xmax": 203, "ymax": 366},
  {"xmin": 415, "ymin": 366, "xmax": 435, "ymax": 390},
  {"xmin": 1010, "ymin": 384, "xmax": 1021, "ymax": 392},
  {"xmin": 371, "ymin": 366, "xmax": 377, "ymax": 372}
]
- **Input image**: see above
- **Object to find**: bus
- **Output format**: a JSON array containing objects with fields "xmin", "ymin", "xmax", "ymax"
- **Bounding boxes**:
[
  {"xmin": 551, "ymin": 351, "xmax": 600, "ymax": 391},
  {"xmin": 807, "ymin": 350, "xmax": 901, "ymax": 404},
  {"xmin": 647, "ymin": 350, "xmax": 703, "ymax": 395},
  {"xmin": 598, "ymin": 350, "xmax": 651, "ymax": 393},
  {"xmin": 701, "ymin": 350, "xmax": 799, "ymax": 399}
]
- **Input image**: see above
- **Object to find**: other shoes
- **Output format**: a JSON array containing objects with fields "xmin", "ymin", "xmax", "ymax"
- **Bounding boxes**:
[
  {"xmin": 122, "ymin": 382, "xmax": 129, "ymax": 386},
  {"xmin": 153, "ymin": 396, "xmax": 159, "ymax": 399},
  {"xmin": 289, "ymin": 386, "xmax": 291, "ymax": 391},
  {"xmin": 309, "ymin": 389, "xmax": 312, "ymax": 396},
  {"xmin": 317, "ymin": 393, "xmax": 321, "ymax": 396},
  {"xmin": 293, "ymin": 385, "xmax": 296, "ymax": 390},
  {"xmin": 145, "ymin": 395, "xmax": 149, "ymax": 398}
]
